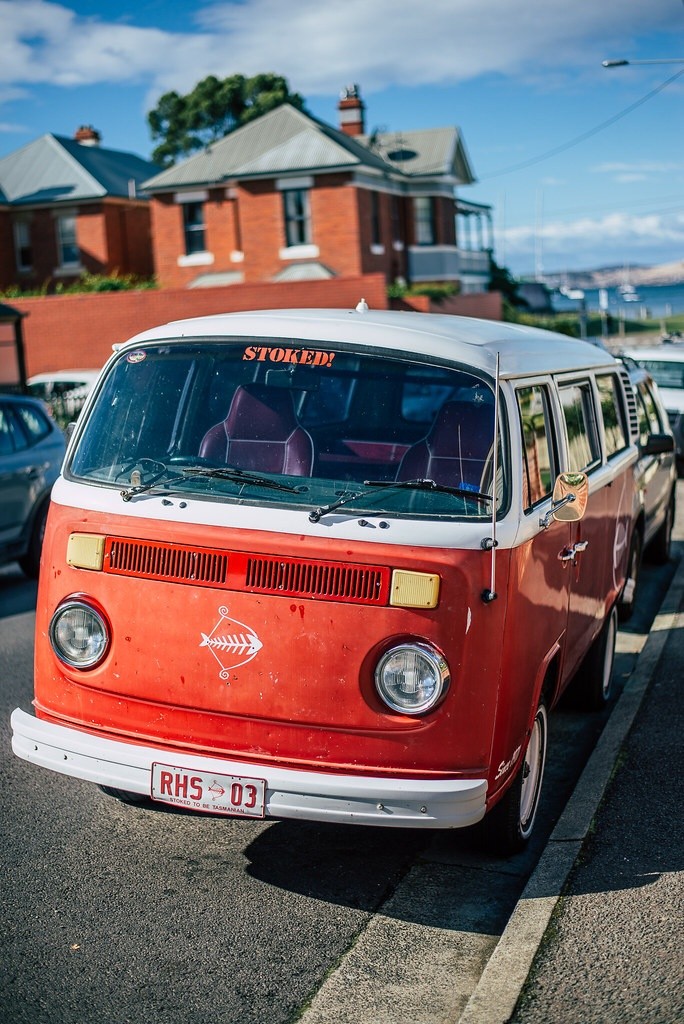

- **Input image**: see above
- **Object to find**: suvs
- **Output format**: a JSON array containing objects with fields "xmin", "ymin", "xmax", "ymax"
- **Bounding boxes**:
[{"xmin": 459, "ymin": 354, "xmax": 678, "ymax": 621}]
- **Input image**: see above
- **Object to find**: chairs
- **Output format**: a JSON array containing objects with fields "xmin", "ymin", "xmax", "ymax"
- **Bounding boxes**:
[
  {"xmin": 198, "ymin": 382, "xmax": 314, "ymax": 480},
  {"xmin": 394, "ymin": 399, "xmax": 501, "ymax": 503}
]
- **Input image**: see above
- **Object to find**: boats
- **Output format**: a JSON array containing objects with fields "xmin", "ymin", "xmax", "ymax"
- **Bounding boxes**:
[{"xmin": 618, "ymin": 285, "xmax": 637, "ymax": 302}]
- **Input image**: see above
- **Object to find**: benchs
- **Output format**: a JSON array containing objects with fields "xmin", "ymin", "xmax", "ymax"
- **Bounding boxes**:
[{"xmin": 314, "ymin": 436, "xmax": 411, "ymax": 485}]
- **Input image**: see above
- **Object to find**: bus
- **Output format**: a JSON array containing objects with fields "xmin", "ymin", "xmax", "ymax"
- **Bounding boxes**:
[{"xmin": 9, "ymin": 296, "xmax": 639, "ymax": 854}]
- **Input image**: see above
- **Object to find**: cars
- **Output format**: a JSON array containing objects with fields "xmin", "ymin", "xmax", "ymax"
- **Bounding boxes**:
[
  {"xmin": 529, "ymin": 385, "xmax": 581, "ymax": 432},
  {"xmin": 402, "ymin": 369, "xmax": 455, "ymax": 423},
  {"xmin": 0, "ymin": 393, "xmax": 67, "ymax": 578},
  {"xmin": 623, "ymin": 343, "xmax": 684, "ymax": 460},
  {"xmin": 25, "ymin": 370, "xmax": 101, "ymax": 417}
]
